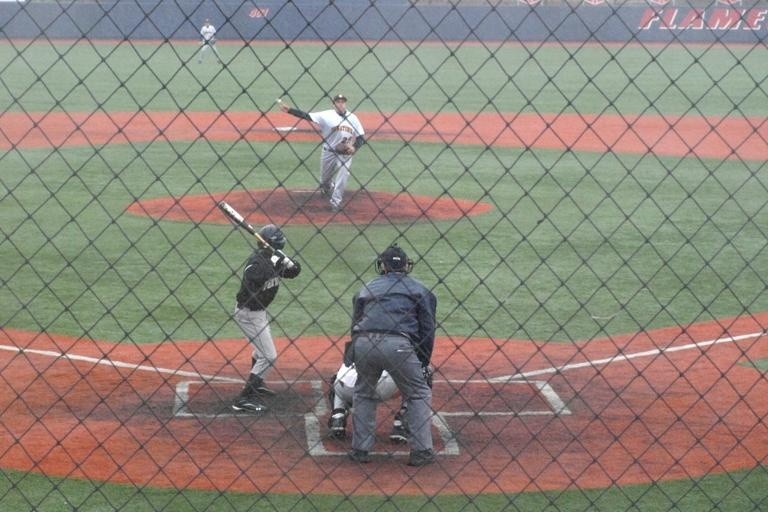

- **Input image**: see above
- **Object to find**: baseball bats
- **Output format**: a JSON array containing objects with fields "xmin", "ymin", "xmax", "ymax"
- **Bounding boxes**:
[
  {"xmin": 218, "ymin": 200, "xmax": 297, "ymax": 270},
  {"xmin": 271, "ymin": 127, "xmax": 321, "ymax": 132}
]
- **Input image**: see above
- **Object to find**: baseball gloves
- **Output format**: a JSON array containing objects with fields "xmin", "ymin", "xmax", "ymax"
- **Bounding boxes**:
[{"xmin": 335, "ymin": 143, "xmax": 355, "ymax": 155}]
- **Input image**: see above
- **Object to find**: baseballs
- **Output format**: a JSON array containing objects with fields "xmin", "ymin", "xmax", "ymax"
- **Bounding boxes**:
[{"xmin": 276, "ymin": 99, "xmax": 281, "ymax": 104}]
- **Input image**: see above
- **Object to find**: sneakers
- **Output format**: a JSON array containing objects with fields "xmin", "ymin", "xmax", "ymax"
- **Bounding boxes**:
[
  {"xmin": 390, "ymin": 420, "xmax": 409, "ymax": 443},
  {"xmin": 217, "ymin": 59, "xmax": 220, "ymax": 63},
  {"xmin": 407, "ymin": 449, "xmax": 434, "ymax": 466},
  {"xmin": 329, "ymin": 413, "xmax": 346, "ymax": 438},
  {"xmin": 257, "ymin": 385, "xmax": 275, "ymax": 394},
  {"xmin": 320, "ymin": 184, "xmax": 334, "ymax": 197},
  {"xmin": 199, "ymin": 60, "xmax": 201, "ymax": 63},
  {"xmin": 232, "ymin": 397, "xmax": 268, "ymax": 412},
  {"xmin": 349, "ymin": 448, "xmax": 370, "ymax": 462},
  {"xmin": 331, "ymin": 205, "xmax": 339, "ymax": 213}
]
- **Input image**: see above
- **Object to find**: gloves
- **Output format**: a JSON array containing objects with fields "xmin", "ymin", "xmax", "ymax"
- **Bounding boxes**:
[
  {"xmin": 269, "ymin": 256, "xmax": 280, "ymax": 267},
  {"xmin": 274, "ymin": 250, "xmax": 294, "ymax": 269}
]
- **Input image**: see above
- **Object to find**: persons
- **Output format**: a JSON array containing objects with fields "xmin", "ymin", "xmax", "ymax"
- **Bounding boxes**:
[
  {"xmin": 274, "ymin": 93, "xmax": 366, "ymax": 209},
  {"xmin": 340, "ymin": 242, "xmax": 443, "ymax": 465},
  {"xmin": 327, "ymin": 357, "xmax": 418, "ymax": 444},
  {"xmin": 230, "ymin": 223, "xmax": 302, "ymax": 413},
  {"xmin": 196, "ymin": 17, "xmax": 222, "ymax": 65}
]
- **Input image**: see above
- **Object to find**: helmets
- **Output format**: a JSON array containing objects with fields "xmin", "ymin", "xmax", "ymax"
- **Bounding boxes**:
[
  {"xmin": 257, "ymin": 225, "xmax": 285, "ymax": 249},
  {"xmin": 376, "ymin": 245, "xmax": 414, "ymax": 273}
]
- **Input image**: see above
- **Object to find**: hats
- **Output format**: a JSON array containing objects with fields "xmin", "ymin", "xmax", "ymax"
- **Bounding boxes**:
[
  {"xmin": 204, "ymin": 19, "xmax": 208, "ymax": 21},
  {"xmin": 334, "ymin": 95, "xmax": 348, "ymax": 103}
]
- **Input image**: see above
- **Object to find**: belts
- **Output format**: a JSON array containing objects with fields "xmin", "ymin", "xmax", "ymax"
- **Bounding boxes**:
[
  {"xmin": 324, "ymin": 147, "xmax": 337, "ymax": 152},
  {"xmin": 359, "ymin": 331, "xmax": 411, "ymax": 334}
]
